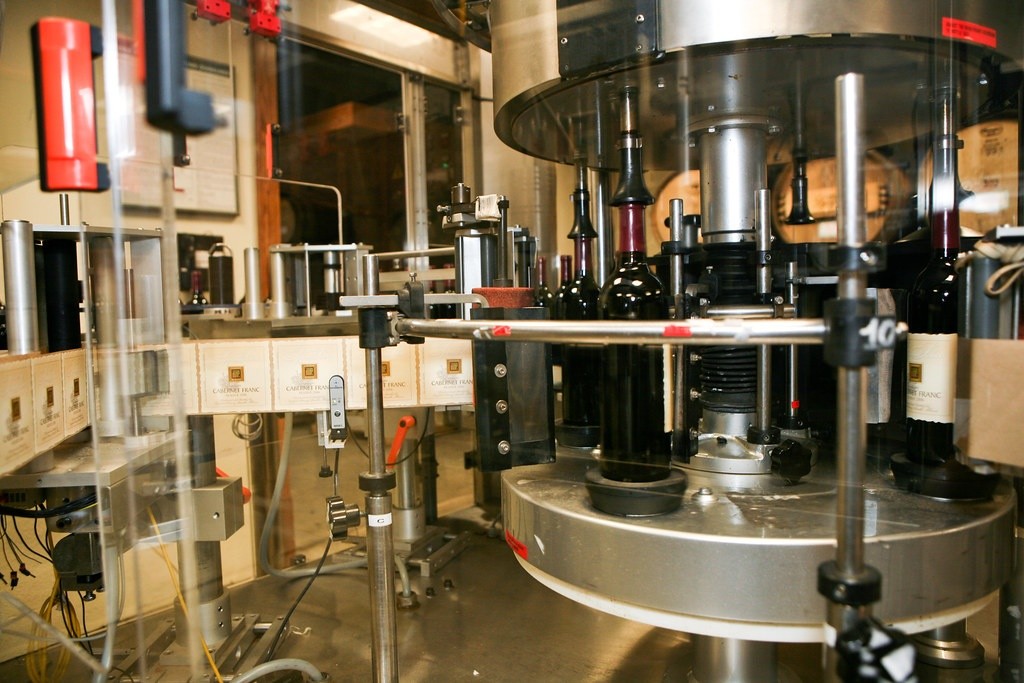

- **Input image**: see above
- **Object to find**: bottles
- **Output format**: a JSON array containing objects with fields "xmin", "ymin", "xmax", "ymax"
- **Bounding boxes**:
[
  {"xmin": 441, "ymin": 263, "xmax": 456, "ymax": 319},
  {"xmin": 186, "ymin": 271, "xmax": 209, "ymax": 313},
  {"xmin": 888, "ymin": 212, "xmax": 990, "ymax": 499},
  {"xmin": 601, "ymin": 204, "xmax": 667, "ymax": 320},
  {"xmin": 533, "ymin": 258, "xmax": 555, "ymax": 307},
  {"xmin": 551, "ymin": 255, "xmax": 572, "ymax": 319},
  {"xmin": 431, "ymin": 265, "xmax": 440, "ymax": 319},
  {"xmin": 563, "ymin": 238, "xmax": 607, "ymax": 431}
]
